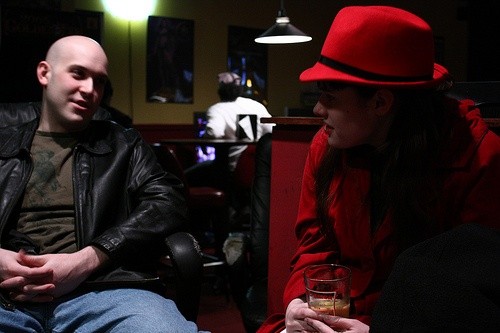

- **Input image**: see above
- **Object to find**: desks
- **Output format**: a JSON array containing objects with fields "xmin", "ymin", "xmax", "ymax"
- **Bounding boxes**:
[{"xmin": 166, "ymin": 138, "xmax": 262, "ymax": 258}]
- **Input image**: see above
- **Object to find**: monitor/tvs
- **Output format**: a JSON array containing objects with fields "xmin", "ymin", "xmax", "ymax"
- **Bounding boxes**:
[{"xmin": 193, "ymin": 111, "xmax": 216, "ymax": 164}]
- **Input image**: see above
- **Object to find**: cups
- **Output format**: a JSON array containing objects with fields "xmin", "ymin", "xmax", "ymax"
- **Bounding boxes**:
[{"xmin": 302, "ymin": 264, "xmax": 351, "ymax": 320}]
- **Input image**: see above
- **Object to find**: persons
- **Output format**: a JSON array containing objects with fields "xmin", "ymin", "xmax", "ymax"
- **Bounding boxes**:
[
  {"xmin": 0, "ymin": 36, "xmax": 212, "ymax": 333},
  {"xmin": 183, "ymin": 72, "xmax": 277, "ymax": 201},
  {"xmin": 254, "ymin": 5, "xmax": 500, "ymax": 333}
]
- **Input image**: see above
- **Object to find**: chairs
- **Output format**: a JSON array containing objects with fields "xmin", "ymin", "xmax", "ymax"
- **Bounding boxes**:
[{"xmin": 151, "ymin": 137, "xmax": 270, "ymax": 332}]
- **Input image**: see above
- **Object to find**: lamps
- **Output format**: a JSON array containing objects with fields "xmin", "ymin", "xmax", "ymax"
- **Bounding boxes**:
[{"xmin": 254, "ymin": 0, "xmax": 313, "ymax": 44}]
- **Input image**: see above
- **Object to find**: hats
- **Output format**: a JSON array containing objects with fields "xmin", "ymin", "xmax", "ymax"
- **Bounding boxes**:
[{"xmin": 299, "ymin": 5, "xmax": 447, "ymax": 83}]
